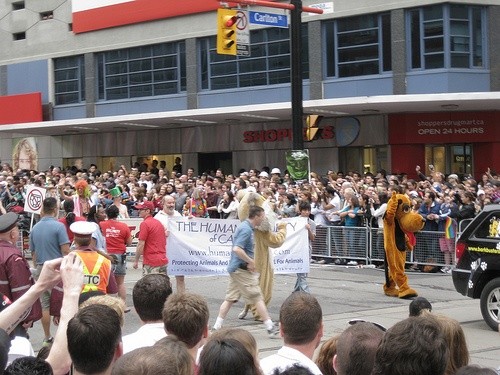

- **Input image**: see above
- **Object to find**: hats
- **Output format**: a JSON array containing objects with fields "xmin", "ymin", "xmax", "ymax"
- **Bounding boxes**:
[
  {"xmin": 484, "ymin": 198, "xmax": 491, "ymax": 204},
  {"xmin": 258, "ymin": 171, "xmax": 268, "ymax": 179},
  {"xmin": 70, "ymin": 220, "xmax": 96, "ymax": 237},
  {"xmin": 135, "ymin": 201, "xmax": 154, "ymax": 211},
  {"xmin": 0, "ymin": 212, "xmax": 21, "ymax": 234},
  {"xmin": 270, "ymin": 168, "xmax": 281, "ymax": 174},
  {"xmin": 447, "ymin": 174, "xmax": 458, "ymax": 181},
  {"xmin": 477, "ymin": 190, "xmax": 484, "ymax": 196},
  {"xmin": 326, "ymin": 186, "xmax": 334, "ymax": 195},
  {"xmin": 321, "ymin": 178, "xmax": 328, "ymax": 187},
  {"xmin": 110, "ymin": 186, "xmax": 123, "ymax": 200}
]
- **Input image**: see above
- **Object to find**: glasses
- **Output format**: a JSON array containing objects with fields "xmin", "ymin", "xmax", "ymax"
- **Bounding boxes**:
[{"xmin": 349, "ymin": 320, "xmax": 387, "ymax": 333}]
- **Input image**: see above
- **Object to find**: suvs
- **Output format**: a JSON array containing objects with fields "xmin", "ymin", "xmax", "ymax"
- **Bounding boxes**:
[{"xmin": 451, "ymin": 202, "xmax": 500, "ymax": 332}]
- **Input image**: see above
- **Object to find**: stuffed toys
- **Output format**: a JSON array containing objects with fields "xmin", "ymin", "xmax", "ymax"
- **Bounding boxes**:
[
  {"xmin": 382, "ymin": 190, "xmax": 426, "ymax": 299},
  {"xmin": 236, "ymin": 187, "xmax": 288, "ymax": 321}
]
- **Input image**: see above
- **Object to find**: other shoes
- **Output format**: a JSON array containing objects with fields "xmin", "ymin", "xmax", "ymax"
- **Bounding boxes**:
[
  {"xmin": 42, "ymin": 335, "xmax": 55, "ymax": 347},
  {"xmin": 311, "ymin": 256, "xmax": 364, "ymax": 266},
  {"xmin": 440, "ymin": 266, "xmax": 453, "ymax": 273},
  {"xmin": 373, "ymin": 261, "xmax": 440, "ymax": 273},
  {"xmin": 124, "ymin": 306, "xmax": 130, "ymax": 313},
  {"xmin": 268, "ymin": 322, "xmax": 280, "ymax": 339}
]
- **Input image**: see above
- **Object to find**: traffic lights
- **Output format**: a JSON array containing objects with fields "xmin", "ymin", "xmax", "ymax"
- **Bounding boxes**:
[
  {"xmin": 216, "ymin": 8, "xmax": 240, "ymax": 55},
  {"xmin": 306, "ymin": 114, "xmax": 323, "ymax": 142}
]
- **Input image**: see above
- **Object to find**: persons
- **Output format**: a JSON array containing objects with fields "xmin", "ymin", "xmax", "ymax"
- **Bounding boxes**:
[
  {"xmin": 58, "ymin": 199, "xmax": 111, "ymax": 259},
  {"xmin": 0, "ymin": 212, "xmax": 43, "ymax": 339},
  {"xmin": 29, "ymin": 197, "xmax": 70, "ymax": 347},
  {"xmin": 291, "ymin": 202, "xmax": 317, "ymax": 295},
  {"xmin": 153, "ymin": 195, "xmax": 185, "ymax": 296},
  {"xmin": 99, "ymin": 205, "xmax": 132, "ymax": 313},
  {"xmin": 133, "ymin": 201, "xmax": 168, "ymax": 277},
  {"xmin": 213, "ymin": 207, "xmax": 279, "ymax": 339},
  {"xmin": 49, "ymin": 221, "xmax": 119, "ymax": 326},
  {"xmin": 0, "ymin": 157, "xmax": 500, "ymax": 273},
  {"xmin": 0, "ymin": 252, "xmax": 498, "ymax": 375}
]
